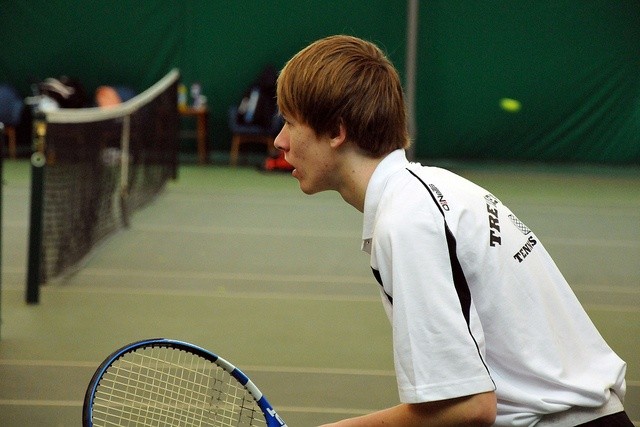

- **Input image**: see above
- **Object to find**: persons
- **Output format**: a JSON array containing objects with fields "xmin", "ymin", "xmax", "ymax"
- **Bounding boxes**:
[
  {"xmin": 274, "ymin": 35, "xmax": 635, "ymax": 427},
  {"xmin": 238, "ymin": 62, "xmax": 279, "ymax": 123},
  {"xmin": 39, "ymin": 76, "xmax": 86, "ymax": 107}
]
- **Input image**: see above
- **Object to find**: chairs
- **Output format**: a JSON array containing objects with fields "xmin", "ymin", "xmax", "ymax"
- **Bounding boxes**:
[
  {"xmin": 0, "ymin": 87, "xmax": 23, "ymax": 159},
  {"xmin": 229, "ymin": 106, "xmax": 284, "ymax": 166}
]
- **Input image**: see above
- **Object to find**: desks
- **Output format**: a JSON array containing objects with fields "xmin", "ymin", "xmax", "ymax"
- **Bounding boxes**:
[{"xmin": 179, "ymin": 102, "xmax": 208, "ymax": 163}]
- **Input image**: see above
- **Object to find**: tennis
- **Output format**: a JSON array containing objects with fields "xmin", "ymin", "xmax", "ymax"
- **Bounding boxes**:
[{"xmin": 500, "ymin": 98, "xmax": 521, "ymax": 113}]
